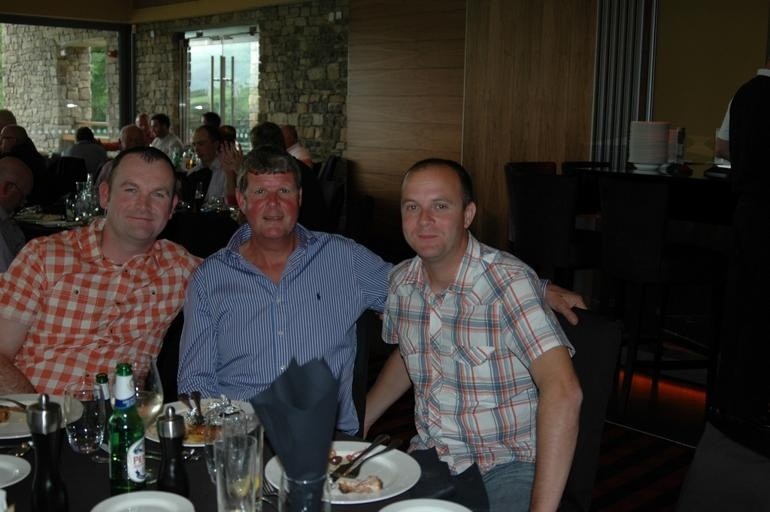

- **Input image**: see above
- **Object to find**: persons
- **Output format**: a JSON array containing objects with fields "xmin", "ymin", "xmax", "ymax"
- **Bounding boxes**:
[
  {"xmin": 201, "ymin": 112, "xmax": 220, "ymax": 140},
  {"xmin": 65, "ymin": 126, "xmax": 106, "ymax": 176},
  {"xmin": 1, "ymin": 147, "xmax": 208, "ymax": 395},
  {"xmin": 715, "ymin": 42, "xmax": 770, "ymax": 439},
  {"xmin": 95, "ymin": 125, "xmax": 146, "ymax": 195},
  {"xmin": 361, "ymin": 157, "xmax": 583, "ymax": 510},
  {"xmin": 178, "ymin": 147, "xmax": 588, "ymax": 439},
  {"xmin": 0, "ymin": 124, "xmax": 33, "ymax": 168},
  {"xmin": 0, "ymin": 109, "xmax": 15, "ymax": 132},
  {"xmin": 151, "ymin": 113, "xmax": 181, "ymax": 159},
  {"xmin": 281, "ymin": 125, "xmax": 311, "ymax": 166},
  {"xmin": 117, "ymin": 114, "xmax": 150, "ymax": 161},
  {"xmin": 219, "ymin": 122, "xmax": 312, "ymax": 230},
  {"xmin": 0, "ymin": 155, "xmax": 34, "ymax": 274},
  {"xmin": 185, "ymin": 126, "xmax": 230, "ymax": 209}
]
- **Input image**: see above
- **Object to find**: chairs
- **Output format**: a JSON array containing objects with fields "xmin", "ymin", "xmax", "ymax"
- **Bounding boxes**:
[
  {"xmin": 157, "ymin": 211, "xmax": 240, "ymax": 258},
  {"xmin": 562, "ymin": 161, "xmax": 636, "ymax": 171},
  {"xmin": 512, "ymin": 173, "xmax": 600, "ymax": 289},
  {"xmin": 505, "ymin": 250, "xmax": 622, "ymax": 512},
  {"xmin": 313, "ymin": 161, "xmax": 324, "ymax": 177},
  {"xmin": 46, "ymin": 156, "xmax": 87, "ymax": 187},
  {"xmin": 180, "ymin": 167, "xmax": 213, "ymax": 210},
  {"xmin": 353, "ymin": 324, "xmax": 379, "ymax": 437},
  {"xmin": 149, "ymin": 328, "xmax": 183, "ymax": 403},
  {"xmin": 299, "ymin": 179, "xmax": 345, "ymax": 232},
  {"xmin": 603, "ymin": 180, "xmax": 730, "ymax": 362},
  {"xmin": 505, "ymin": 162, "xmax": 556, "ymax": 264}
]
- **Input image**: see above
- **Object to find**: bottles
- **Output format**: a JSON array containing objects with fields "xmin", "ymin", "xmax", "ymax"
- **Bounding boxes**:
[
  {"xmin": 109, "ymin": 364, "xmax": 147, "ymax": 496},
  {"xmin": 27, "ymin": 394, "xmax": 68, "ymax": 512},
  {"xmin": 155, "ymin": 407, "xmax": 188, "ymax": 499},
  {"xmin": 92, "ymin": 373, "xmax": 115, "ymax": 460}
]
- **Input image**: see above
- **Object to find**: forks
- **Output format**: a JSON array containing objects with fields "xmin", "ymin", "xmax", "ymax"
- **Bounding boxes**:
[
  {"xmin": 262, "ymin": 479, "xmax": 280, "ymax": 497},
  {"xmin": 334, "ymin": 442, "xmax": 402, "ymax": 479}
]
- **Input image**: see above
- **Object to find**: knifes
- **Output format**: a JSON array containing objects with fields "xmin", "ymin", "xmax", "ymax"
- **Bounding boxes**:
[{"xmin": 329, "ymin": 434, "xmax": 387, "ymax": 485}]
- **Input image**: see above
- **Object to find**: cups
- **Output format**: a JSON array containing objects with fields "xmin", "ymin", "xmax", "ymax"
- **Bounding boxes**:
[
  {"xmin": 213, "ymin": 435, "xmax": 260, "ymax": 512},
  {"xmin": 276, "ymin": 472, "xmax": 332, "ymax": 512},
  {"xmin": 109, "ymin": 352, "xmax": 164, "ymax": 437},
  {"xmin": 89, "ymin": 489, "xmax": 195, "ymax": 512},
  {"xmin": 62, "ymin": 381, "xmax": 106, "ymax": 454}
]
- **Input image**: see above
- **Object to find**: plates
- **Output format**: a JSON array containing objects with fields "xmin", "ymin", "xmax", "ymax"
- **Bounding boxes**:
[
  {"xmin": 376, "ymin": 498, "xmax": 472, "ymax": 512},
  {"xmin": 145, "ymin": 398, "xmax": 259, "ymax": 447},
  {"xmin": 0, "ymin": 453, "xmax": 32, "ymax": 490},
  {"xmin": 265, "ymin": 440, "xmax": 421, "ymax": 504},
  {"xmin": 0, "ymin": 392, "xmax": 84, "ymax": 439}
]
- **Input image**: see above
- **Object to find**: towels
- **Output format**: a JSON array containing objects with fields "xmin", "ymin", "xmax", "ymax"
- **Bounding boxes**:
[
  {"xmin": 251, "ymin": 356, "xmax": 342, "ymax": 511},
  {"xmin": 181, "ymin": 168, "xmax": 212, "ymax": 203}
]
